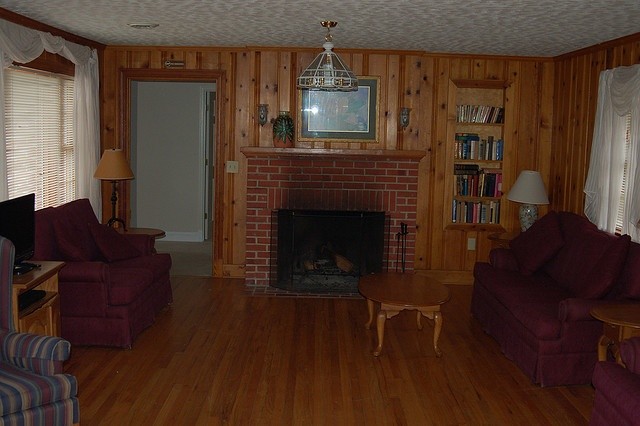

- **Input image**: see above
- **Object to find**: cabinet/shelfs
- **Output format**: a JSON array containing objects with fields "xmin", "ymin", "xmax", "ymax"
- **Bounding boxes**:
[
  {"xmin": 9, "ymin": 260, "xmax": 67, "ymax": 343},
  {"xmin": 428, "ymin": 55, "xmax": 539, "ymax": 286}
]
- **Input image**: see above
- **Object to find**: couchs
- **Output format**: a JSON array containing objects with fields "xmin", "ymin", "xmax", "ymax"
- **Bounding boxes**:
[
  {"xmin": 34, "ymin": 198, "xmax": 172, "ymax": 350},
  {"xmin": 590, "ymin": 336, "xmax": 640, "ymax": 426},
  {"xmin": 471, "ymin": 211, "xmax": 640, "ymax": 388},
  {"xmin": 0, "ymin": 236, "xmax": 80, "ymax": 426}
]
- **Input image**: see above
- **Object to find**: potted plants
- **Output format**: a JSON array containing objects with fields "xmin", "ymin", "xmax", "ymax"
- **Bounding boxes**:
[{"xmin": 272, "ymin": 116, "xmax": 294, "ymax": 148}]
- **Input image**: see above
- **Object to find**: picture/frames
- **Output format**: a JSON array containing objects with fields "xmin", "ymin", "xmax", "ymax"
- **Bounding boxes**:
[{"xmin": 297, "ymin": 76, "xmax": 382, "ymax": 144}]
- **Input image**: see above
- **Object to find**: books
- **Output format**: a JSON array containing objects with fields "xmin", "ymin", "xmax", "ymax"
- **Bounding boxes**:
[
  {"xmin": 454, "ymin": 164, "xmax": 502, "ymax": 198},
  {"xmin": 452, "ymin": 201, "xmax": 499, "ymax": 226},
  {"xmin": 457, "ymin": 104, "xmax": 504, "ymax": 123},
  {"xmin": 454, "ymin": 132, "xmax": 503, "ymax": 161}
]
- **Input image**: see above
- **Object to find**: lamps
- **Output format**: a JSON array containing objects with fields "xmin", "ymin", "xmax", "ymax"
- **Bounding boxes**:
[
  {"xmin": 255, "ymin": 103, "xmax": 268, "ymax": 127},
  {"xmin": 399, "ymin": 107, "xmax": 411, "ymax": 135},
  {"xmin": 296, "ymin": 21, "xmax": 358, "ymax": 93},
  {"xmin": 504, "ymin": 169, "xmax": 548, "ymax": 234},
  {"xmin": 93, "ymin": 149, "xmax": 135, "ymax": 232}
]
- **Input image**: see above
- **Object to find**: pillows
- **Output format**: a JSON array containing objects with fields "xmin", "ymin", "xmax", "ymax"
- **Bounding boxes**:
[
  {"xmin": 576, "ymin": 234, "xmax": 631, "ymax": 299},
  {"xmin": 56, "ymin": 235, "xmax": 94, "ymax": 263},
  {"xmin": 510, "ymin": 211, "xmax": 564, "ymax": 279},
  {"xmin": 87, "ymin": 223, "xmax": 141, "ymax": 262}
]
